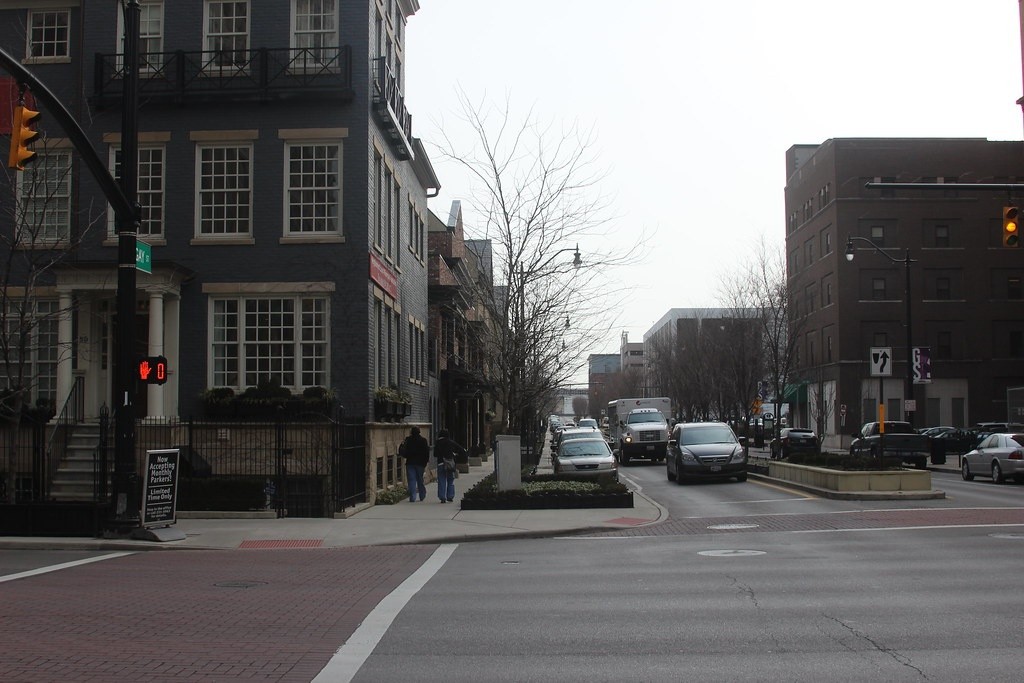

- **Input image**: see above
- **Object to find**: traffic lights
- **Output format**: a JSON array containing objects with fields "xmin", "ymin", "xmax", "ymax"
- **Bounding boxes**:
[
  {"xmin": 8, "ymin": 107, "xmax": 42, "ymax": 171},
  {"xmin": 139, "ymin": 355, "xmax": 169, "ymax": 385},
  {"xmin": 1003, "ymin": 207, "xmax": 1019, "ymax": 248}
]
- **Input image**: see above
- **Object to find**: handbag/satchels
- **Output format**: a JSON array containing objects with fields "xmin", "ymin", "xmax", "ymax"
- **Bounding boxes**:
[
  {"xmin": 399, "ymin": 438, "xmax": 409, "ymax": 458},
  {"xmin": 443, "ymin": 457, "xmax": 456, "ymax": 473}
]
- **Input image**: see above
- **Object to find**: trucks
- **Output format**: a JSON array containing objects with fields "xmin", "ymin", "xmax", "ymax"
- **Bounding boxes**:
[{"xmin": 607, "ymin": 397, "xmax": 673, "ymax": 466}]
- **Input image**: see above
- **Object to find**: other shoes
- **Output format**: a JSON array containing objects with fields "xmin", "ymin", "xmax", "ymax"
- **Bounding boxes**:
[
  {"xmin": 447, "ymin": 498, "xmax": 453, "ymax": 502},
  {"xmin": 441, "ymin": 500, "xmax": 445, "ymax": 503}
]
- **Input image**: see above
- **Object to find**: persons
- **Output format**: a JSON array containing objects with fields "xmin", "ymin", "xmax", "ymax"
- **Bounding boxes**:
[
  {"xmin": 433, "ymin": 430, "xmax": 468, "ymax": 503},
  {"xmin": 399, "ymin": 427, "xmax": 430, "ymax": 502}
]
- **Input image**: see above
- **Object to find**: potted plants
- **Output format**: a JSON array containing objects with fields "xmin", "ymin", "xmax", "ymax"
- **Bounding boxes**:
[
  {"xmin": 374, "ymin": 384, "xmax": 412, "ymax": 423},
  {"xmin": 478, "ymin": 442, "xmax": 487, "ymax": 462},
  {"xmin": 375, "ymin": 484, "xmax": 409, "ymax": 505},
  {"xmin": 467, "ymin": 446, "xmax": 482, "ymax": 466},
  {"xmin": 455, "ymin": 452, "xmax": 469, "ymax": 473},
  {"xmin": 177, "ymin": 378, "xmax": 343, "ymax": 423}
]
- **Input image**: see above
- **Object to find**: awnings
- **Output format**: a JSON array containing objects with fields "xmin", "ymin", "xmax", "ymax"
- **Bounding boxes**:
[{"xmin": 771, "ymin": 384, "xmax": 807, "ymax": 403}]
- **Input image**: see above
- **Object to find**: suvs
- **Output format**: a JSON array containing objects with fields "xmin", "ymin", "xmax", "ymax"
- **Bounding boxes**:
[{"xmin": 769, "ymin": 427, "xmax": 822, "ymax": 460}]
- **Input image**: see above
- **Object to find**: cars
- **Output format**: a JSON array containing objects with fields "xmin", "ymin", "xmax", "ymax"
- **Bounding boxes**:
[
  {"xmin": 550, "ymin": 437, "xmax": 619, "ymax": 482},
  {"xmin": 548, "ymin": 412, "xmax": 616, "ymax": 454},
  {"xmin": 914, "ymin": 420, "xmax": 1024, "ymax": 455},
  {"xmin": 672, "ymin": 415, "xmax": 788, "ymax": 440},
  {"xmin": 961, "ymin": 431, "xmax": 1024, "ymax": 485},
  {"xmin": 665, "ymin": 422, "xmax": 749, "ymax": 485}
]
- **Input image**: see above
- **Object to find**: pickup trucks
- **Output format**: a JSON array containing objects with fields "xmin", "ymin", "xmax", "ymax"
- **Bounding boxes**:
[{"xmin": 850, "ymin": 420, "xmax": 931, "ymax": 470}]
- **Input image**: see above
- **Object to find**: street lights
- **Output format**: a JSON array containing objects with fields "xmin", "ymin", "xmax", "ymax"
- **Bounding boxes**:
[
  {"xmin": 843, "ymin": 230, "xmax": 918, "ymax": 425},
  {"xmin": 515, "ymin": 242, "xmax": 583, "ymax": 444}
]
